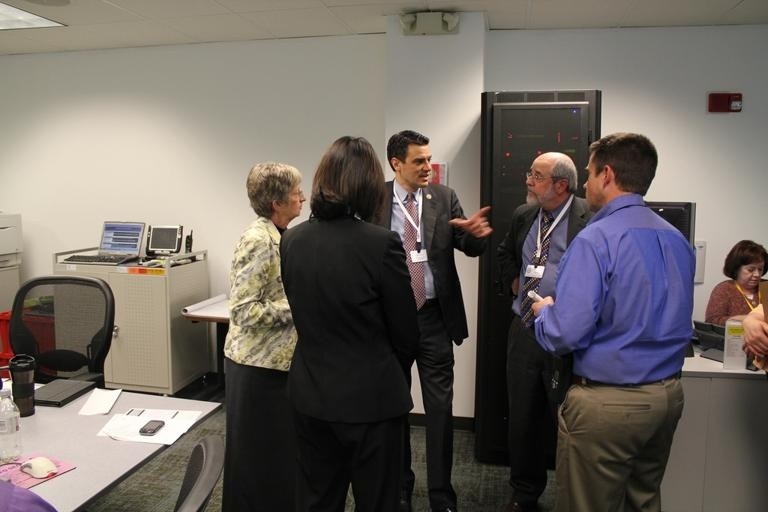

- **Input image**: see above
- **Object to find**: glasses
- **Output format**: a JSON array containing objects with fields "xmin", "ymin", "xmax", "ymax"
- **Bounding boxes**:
[{"xmin": 525, "ymin": 171, "xmax": 565, "ymax": 183}]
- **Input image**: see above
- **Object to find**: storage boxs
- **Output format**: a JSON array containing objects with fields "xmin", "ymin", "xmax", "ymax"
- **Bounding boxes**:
[
  {"xmin": 0, "ymin": 307, "xmax": 55, "ymax": 353},
  {"xmin": 0, "ymin": 351, "xmax": 57, "ymax": 378}
]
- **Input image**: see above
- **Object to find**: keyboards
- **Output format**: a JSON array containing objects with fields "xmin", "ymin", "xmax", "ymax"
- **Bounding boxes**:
[{"xmin": 63, "ymin": 253, "xmax": 132, "ymax": 267}]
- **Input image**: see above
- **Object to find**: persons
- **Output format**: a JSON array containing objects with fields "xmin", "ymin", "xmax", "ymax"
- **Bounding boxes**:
[
  {"xmin": 705, "ymin": 239, "xmax": 768, "ymax": 331},
  {"xmin": 370, "ymin": 130, "xmax": 493, "ymax": 512},
  {"xmin": 742, "ymin": 302, "xmax": 768, "ymax": 377},
  {"xmin": 222, "ymin": 162, "xmax": 304, "ymax": 512},
  {"xmin": 531, "ymin": 132, "xmax": 696, "ymax": 512},
  {"xmin": 279, "ymin": 135, "xmax": 421, "ymax": 512},
  {"xmin": 496, "ymin": 151, "xmax": 596, "ymax": 512}
]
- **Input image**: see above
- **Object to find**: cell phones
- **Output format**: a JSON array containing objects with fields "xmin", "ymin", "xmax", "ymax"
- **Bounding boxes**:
[{"xmin": 139, "ymin": 420, "xmax": 165, "ymax": 434}]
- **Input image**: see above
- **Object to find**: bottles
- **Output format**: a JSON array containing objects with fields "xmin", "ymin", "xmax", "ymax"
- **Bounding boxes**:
[{"xmin": 1, "ymin": 388, "xmax": 23, "ymax": 461}]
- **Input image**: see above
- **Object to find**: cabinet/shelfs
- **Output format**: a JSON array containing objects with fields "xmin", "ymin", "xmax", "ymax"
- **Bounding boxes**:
[
  {"xmin": 0, "ymin": 266, "xmax": 21, "ymax": 313},
  {"xmin": 53, "ymin": 247, "xmax": 213, "ymax": 395}
]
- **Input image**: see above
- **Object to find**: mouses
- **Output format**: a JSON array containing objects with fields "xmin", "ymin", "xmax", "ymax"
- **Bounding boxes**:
[{"xmin": 20, "ymin": 456, "xmax": 58, "ymax": 479}]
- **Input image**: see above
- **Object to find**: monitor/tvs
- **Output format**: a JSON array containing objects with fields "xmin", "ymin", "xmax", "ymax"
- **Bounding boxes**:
[
  {"xmin": 649, "ymin": 200, "xmax": 695, "ymax": 248},
  {"xmin": 98, "ymin": 221, "xmax": 149, "ymax": 259},
  {"xmin": 148, "ymin": 225, "xmax": 181, "ymax": 254}
]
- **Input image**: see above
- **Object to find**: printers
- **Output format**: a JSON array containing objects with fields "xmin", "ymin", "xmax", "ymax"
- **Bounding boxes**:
[{"xmin": 1, "ymin": 210, "xmax": 22, "ymax": 270}]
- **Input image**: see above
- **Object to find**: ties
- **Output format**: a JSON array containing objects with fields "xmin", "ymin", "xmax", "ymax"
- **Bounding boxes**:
[
  {"xmin": 404, "ymin": 194, "xmax": 426, "ymax": 311},
  {"xmin": 520, "ymin": 213, "xmax": 554, "ymax": 330}
]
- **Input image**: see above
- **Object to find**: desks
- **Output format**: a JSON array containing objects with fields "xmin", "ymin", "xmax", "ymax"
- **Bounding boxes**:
[
  {"xmin": 182, "ymin": 314, "xmax": 230, "ymax": 373},
  {"xmin": 0, "ymin": 377, "xmax": 222, "ymax": 512},
  {"xmin": 661, "ymin": 346, "xmax": 768, "ymax": 512}
]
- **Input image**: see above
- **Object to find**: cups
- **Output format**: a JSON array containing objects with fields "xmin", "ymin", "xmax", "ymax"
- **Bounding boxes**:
[{"xmin": 7, "ymin": 353, "xmax": 36, "ymax": 418}]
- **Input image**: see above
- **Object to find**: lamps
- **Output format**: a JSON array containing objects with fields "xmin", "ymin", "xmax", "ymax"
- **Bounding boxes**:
[{"xmin": 401, "ymin": 12, "xmax": 460, "ymax": 34}]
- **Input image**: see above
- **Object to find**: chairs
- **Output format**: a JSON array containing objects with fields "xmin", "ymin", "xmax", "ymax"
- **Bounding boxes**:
[
  {"xmin": 173, "ymin": 435, "xmax": 226, "ymax": 512},
  {"xmin": 0, "ymin": 276, "xmax": 115, "ymax": 388}
]
